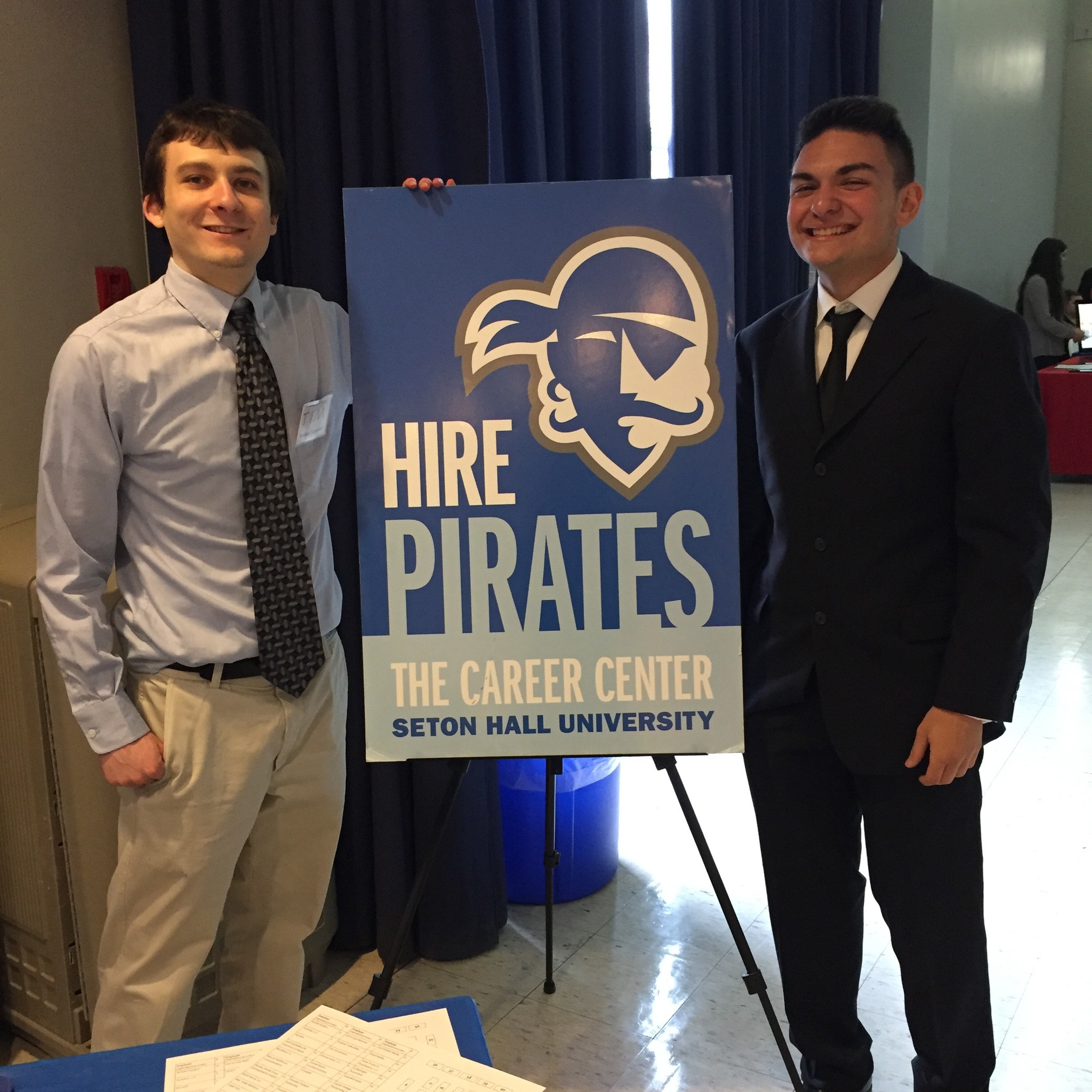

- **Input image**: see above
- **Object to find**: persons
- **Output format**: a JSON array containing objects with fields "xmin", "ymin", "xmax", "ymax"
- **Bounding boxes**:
[
  {"xmin": 33, "ymin": 104, "xmax": 455, "ymax": 1054},
  {"xmin": 1014, "ymin": 238, "xmax": 1086, "ymax": 406},
  {"xmin": 734, "ymin": 98, "xmax": 1052, "ymax": 1092},
  {"xmin": 1063, "ymin": 287, "xmax": 1083, "ymax": 357}
]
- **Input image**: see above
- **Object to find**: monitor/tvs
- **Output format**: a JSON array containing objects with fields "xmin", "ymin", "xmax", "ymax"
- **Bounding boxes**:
[{"xmin": 1075, "ymin": 300, "xmax": 1092, "ymax": 353}]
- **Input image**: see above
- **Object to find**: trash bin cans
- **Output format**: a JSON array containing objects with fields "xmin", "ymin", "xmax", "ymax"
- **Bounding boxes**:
[{"xmin": 496, "ymin": 757, "xmax": 621, "ymax": 906}]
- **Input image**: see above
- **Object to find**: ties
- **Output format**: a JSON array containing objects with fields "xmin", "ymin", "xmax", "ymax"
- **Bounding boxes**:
[
  {"xmin": 225, "ymin": 301, "xmax": 327, "ymax": 699},
  {"xmin": 815, "ymin": 305, "xmax": 865, "ymax": 425}
]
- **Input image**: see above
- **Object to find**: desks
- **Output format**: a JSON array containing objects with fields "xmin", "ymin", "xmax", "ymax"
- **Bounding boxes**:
[{"xmin": 1035, "ymin": 347, "xmax": 1092, "ymax": 488}]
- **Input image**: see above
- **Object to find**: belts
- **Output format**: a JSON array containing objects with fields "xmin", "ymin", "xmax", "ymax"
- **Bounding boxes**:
[{"xmin": 166, "ymin": 628, "xmax": 337, "ymax": 680}]
